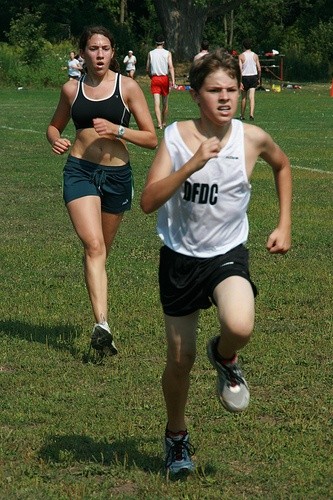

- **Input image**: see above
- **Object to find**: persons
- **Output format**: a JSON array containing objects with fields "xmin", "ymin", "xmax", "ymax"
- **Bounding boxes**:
[
  {"xmin": 123, "ymin": 50, "xmax": 136, "ymax": 79},
  {"xmin": 141, "ymin": 48, "xmax": 292, "ymax": 478},
  {"xmin": 194, "ymin": 41, "xmax": 210, "ymax": 62},
  {"xmin": 146, "ymin": 36, "xmax": 175, "ymax": 129},
  {"xmin": 61, "ymin": 51, "xmax": 87, "ymax": 80},
  {"xmin": 238, "ymin": 41, "xmax": 261, "ymax": 122},
  {"xmin": 47, "ymin": 26, "xmax": 157, "ymax": 358}
]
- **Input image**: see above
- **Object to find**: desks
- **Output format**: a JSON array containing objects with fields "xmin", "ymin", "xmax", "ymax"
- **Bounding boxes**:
[{"xmin": 258, "ymin": 54, "xmax": 285, "ymax": 80}]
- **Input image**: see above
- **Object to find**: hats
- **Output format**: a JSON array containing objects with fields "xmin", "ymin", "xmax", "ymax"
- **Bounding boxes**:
[{"xmin": 155, "ymin": 34, "xmax": 165, "ymax": 45}]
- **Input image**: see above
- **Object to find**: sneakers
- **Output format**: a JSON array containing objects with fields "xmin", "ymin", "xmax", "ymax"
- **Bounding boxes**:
[
  {"xmin": 163, "ymin": 429, "xmax": 196, "ymax": 481},
  {"xmin": 206, "ymin": 334, "xmax": 251, "ymax": 413},
  {"xmin": 91, "ymin": 320, "xmax": 119, "ymax": 357}
]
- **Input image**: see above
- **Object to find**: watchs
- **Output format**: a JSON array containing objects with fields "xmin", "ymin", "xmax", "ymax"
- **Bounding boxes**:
[{"xmin": 116, "ymin": 125, "xmax": 125, "ymax": 139}]
